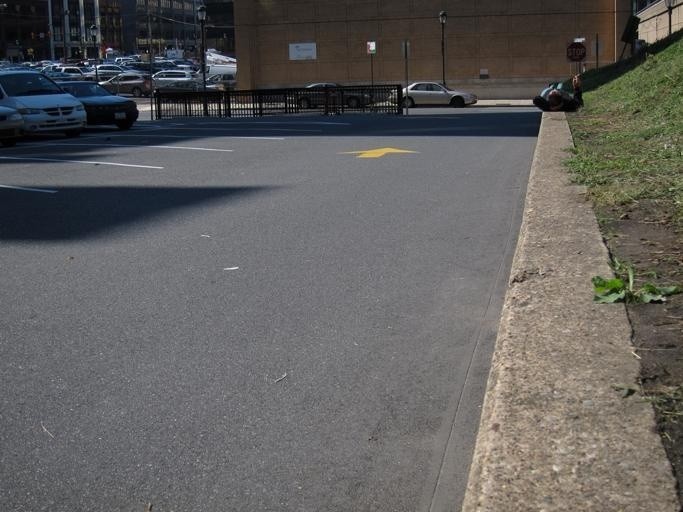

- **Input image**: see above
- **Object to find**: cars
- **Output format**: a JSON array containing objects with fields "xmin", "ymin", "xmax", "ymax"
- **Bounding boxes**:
[
  {"xmin": 388, "ymin": 81, "xmax": 477, "ymax": 108},
  {"xmin": 0, "ymin": 51, "xmax": 238, "ymax": 148},
  {"xmin": 293, "ymin": 82, "xmax": 372, "ymax": 109}
]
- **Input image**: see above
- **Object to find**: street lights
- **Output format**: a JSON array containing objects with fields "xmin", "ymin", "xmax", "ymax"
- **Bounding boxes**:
[
  {"xmin": 90, "ymin": 24, "xmax": 98, "ymax": 91},
  {"xmin": 665, "ymin": 0, "xmax": 677, "ymax": 37},
  {"xmin": 438, "ymin": 9, "xmax": 448, "ymax": 85},
  {"xmin": 197, "ymin": 5, "xmax": 211, "ymax": 116}
]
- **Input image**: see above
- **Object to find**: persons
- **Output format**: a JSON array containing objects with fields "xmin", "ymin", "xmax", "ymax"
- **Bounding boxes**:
[{"xmin": 532, "ymin": 73, "xmax": 584, "ymax": 114}]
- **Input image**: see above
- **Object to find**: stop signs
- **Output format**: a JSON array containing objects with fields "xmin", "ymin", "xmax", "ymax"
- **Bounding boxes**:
[{"xmin": 566, "ymin": 42, "xmax": 586, "ymax": 62}]
